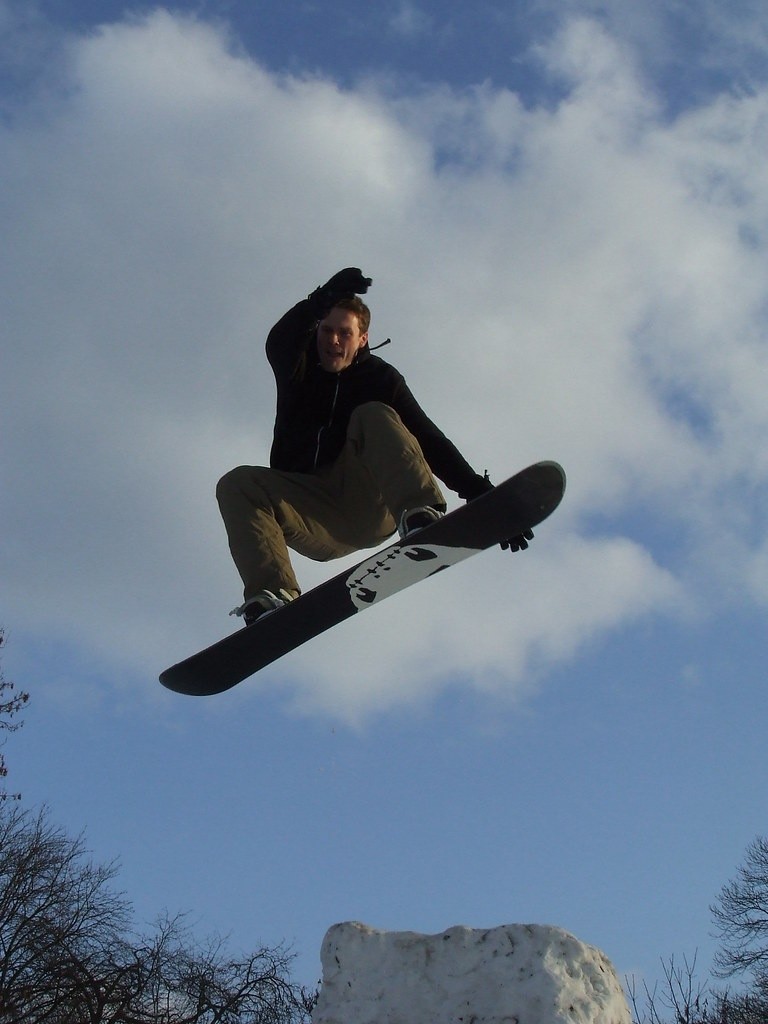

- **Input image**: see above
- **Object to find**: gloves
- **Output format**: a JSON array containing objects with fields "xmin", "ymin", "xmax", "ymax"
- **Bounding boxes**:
[
  {"xmin": 312, "ymin": 268, "xmax": 372, "ymax": 322},
  {"xmin": 458, "ymin": 475, "xmax": 534, "ymax": 553}
]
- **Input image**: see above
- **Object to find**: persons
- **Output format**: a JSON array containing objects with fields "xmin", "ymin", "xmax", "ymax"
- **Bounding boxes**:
[{"xmin": 218, "ymin": 265, "xmax": 537, "ymax": 629}]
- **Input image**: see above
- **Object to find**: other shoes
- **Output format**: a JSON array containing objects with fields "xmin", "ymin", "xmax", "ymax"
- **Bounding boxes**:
[
  {"xmin": 242, "ymin": 590, "xmax": 300, "ymax": 619},
  {"xmin": 402, "ymin": 509, "xmax": 437, "ymax": 531}
]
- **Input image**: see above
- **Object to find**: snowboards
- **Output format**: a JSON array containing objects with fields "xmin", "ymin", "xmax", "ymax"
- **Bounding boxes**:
[{"xmin": 160, "ymin": 459, "xmax": 564, "ymax": 696}]
